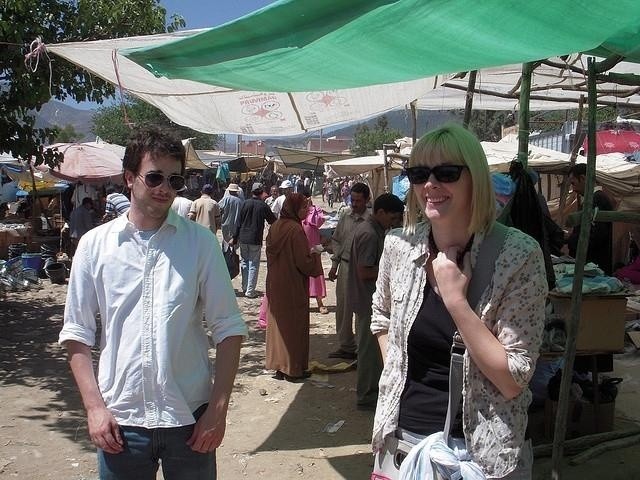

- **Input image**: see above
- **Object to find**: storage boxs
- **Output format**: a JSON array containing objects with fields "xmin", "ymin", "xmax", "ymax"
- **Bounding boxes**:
[
  {"xmin": 549, "ymin": 295, "xmax": 628, "ymax": 354},
  {"xmin": 525, "ymin": 401, "xmax": 616, "ymax": 448}
]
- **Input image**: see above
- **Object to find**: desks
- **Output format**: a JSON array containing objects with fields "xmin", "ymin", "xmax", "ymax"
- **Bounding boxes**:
[{"xmin": 528, "ymin": 348, "xmax": 638, "ymax": 444}]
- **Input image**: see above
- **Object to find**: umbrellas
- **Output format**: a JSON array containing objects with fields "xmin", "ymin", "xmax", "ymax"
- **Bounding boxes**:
[{"xmin": 30, "ymin": 143, "xmax": 124, "ymax": 208}]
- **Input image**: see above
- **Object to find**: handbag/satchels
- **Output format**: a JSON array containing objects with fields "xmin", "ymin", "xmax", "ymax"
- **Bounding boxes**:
[
  {"xmin": 224, "ymin": 246, "xmax": 240, "ymax": 280},
  {"xmin": 371, "ymin": 437, "xmax": 448, "ymax": 480}
]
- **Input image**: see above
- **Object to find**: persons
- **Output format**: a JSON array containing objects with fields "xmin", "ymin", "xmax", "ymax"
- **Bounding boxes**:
[
  {"xmin": 366, "ymin": 120, "xmax": 553, "ymax": 478},
  {"xmin": 69, "ymin": 197, "xmax": 95, "ymax": 245},
  {"xmin": 228, "ymin": 183, "xmax": 276, "ymax": 298},
  {"xmin": 266, "ymin": 194, "xmax": 323, "ymax": 380},
  {"xmin": 248, "ymin": 169, "xmax": 366, "ymax": 219},
  {"xmin": 345, "ymin": 194, "xmax": 404, "ymax": 411},
  {"xmin": 57, "ymin": 126, "xmax": 248, "ymax": 480},
  {"xmin": 211, "ymin": 175, "xmax": 247, "ymax": 197},
  {"xmin": 217, "ymin": 184, "xmax": 244, "ymax": 253},
  {"xmin": 99, "ymin": 186, "xmax": 131, "ymax": 222},
  {"xmin": 328, "ymin": 182, "xmax": 370, "ymax": 360},
  {"xmin": 299, "ymin": 191, "xmax": 329, "ymax": 314},
  {"xmin": 563, "ymin": 164, "xmax": 615, "ymax": 282},
  {"xmin": 187, "ymin": 184, "xmax": 221, "ymax": 235},
  {"xmin": 171, "ymin": 185, "xmax": 193, "ymax": 219}
]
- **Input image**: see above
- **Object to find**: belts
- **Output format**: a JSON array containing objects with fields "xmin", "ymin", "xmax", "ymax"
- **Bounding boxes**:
[{"xmin": 340, "ymin": 257, "xmax": 348, "ymax": 262}]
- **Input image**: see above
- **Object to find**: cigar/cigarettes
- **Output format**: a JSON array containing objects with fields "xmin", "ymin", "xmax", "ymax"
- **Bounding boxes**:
[{"xmin": 324, "ymin": 278, "xmax": 330, "ymax": 280}]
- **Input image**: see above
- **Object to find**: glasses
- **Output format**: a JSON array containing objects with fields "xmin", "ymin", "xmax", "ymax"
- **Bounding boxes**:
[
  {"xmin": 406, "ymin": 165, "xmax": 467, "ymax": 183},
  {"xmin": 135, "ymin": 171, "xmax": 185, "ymax": 190}
]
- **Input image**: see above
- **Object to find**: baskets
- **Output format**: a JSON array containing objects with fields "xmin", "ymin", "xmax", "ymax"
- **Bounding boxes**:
[
  {"xmin": 545, "ymin": 398, "xmax": 589, "ymax": 442},
  {"xmin": 590, "ymin": 403, "xmax": 615, "ymax": 434},
  {"xmin": 528, "ymin": 410, "xmax": 545, "ymax": 444}
]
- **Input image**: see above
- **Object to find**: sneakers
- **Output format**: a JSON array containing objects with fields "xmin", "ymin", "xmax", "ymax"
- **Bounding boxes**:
[
  {"xmin": 328, "ymin": 349, "xmax": 354, "ymax": 359},
  {"xmin": 318, "ymin": 306, "xmax": 328, "ymax": 314}
]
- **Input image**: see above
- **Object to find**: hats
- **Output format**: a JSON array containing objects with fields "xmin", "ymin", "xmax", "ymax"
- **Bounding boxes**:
[
  {"xmin": 251, "ymin": 183, "xmax": 266, "ymax": 190},
  {"xmin": 226, "ymin": 184, "xmax": 240, "ymax": 191},
  {"xmin": 278, "ymin": 179, "xmax": 294, "ymax": 189}
]
